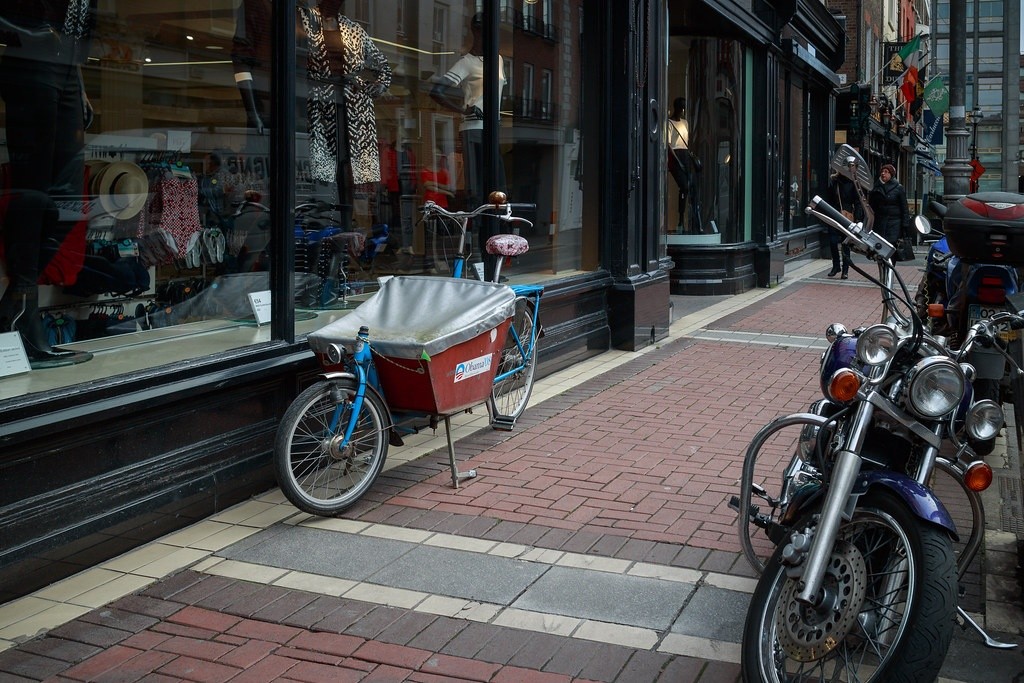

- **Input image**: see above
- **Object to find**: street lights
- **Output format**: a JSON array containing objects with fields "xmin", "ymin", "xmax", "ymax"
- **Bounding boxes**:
[{"xmin": 966, "ymin": 105, "xmax": 984, "ymax": 194}]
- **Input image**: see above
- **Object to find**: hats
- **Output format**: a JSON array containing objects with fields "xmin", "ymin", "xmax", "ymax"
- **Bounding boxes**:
[
  {"xmin": 90, "ymin": 160, "xmax": 149, "ymax": 220},
  {"xmin": 880, "ymin": 164, "xmax": 896, "ymax": 177}
]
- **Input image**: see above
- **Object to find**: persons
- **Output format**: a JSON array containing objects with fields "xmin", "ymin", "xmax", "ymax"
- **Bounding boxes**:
[
  {"xmin": 869, "ymin": 163, "xmax": 910, "ymax": 266},
  {"xmin": 446, "ymin": 136, "xmax": 467, "ymax": 213},
  {"xmin": 204, "ymin": 153, "xmax": 271, "ymax": 279},
  {"xmin": 229, "ymin": 0, "xmax": 277, "ymax": 323},
  {"xmin": 299, "ymin": 0, "xmax": 392, "ymax": 307},
  {"xmin": 421, "ymin": 147, "xmax": 455, "ymax": 275},
  {"xmin": 824, "ymin": 163, "xmax": 863, "ymax": 278},
  {"xmin": 381, "ymin": 126, "xmax": 417, "ymax": 256},
  {"xmin": 0, "ymin": 0, "xmax": 96, "ymax": 361},
  {"xmin": 428, "ymin": 11, "xmax": 509, "ymax": 284},
  {"xmin": 667, "ymin": 96, "xmax": 705, "ymax": 233}
]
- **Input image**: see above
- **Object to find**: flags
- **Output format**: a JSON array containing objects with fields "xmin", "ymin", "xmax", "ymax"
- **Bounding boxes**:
[
  {"xmin": 909, "ymin": 67, "xmax": 926, "ymax": 124},
  {"xmin": 900, "ymin": 35, "xmax": 920, "ymax": 83},
  {"xmin": 898, "ymin": 66, "xmax": 918, "ymax": 103},
  {"xmin": 923, "ymin": 77, "xmax": 950, "ymax": 118}
]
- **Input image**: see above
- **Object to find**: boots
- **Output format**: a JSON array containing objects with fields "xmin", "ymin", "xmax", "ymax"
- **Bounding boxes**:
[
  {"xmin": 827, "ymin": 253, "xmax": 842, "ymax": 276},
  {"xmin": 840, "ymin": 246, "xmax": 850, "ymax": 279}
]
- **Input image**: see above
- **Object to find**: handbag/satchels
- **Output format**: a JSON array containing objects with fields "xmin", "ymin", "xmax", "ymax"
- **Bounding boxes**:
[
  {"xmin": 226, "ymin": 229, "xmax": 249, "ymax": 257},
  {"xmin": 895, "ymin": 237, "xmax": 915, "ymax": 261},
  {"xmin": 839, "ymin": 210, "xmax": 853, "ymax": 222},
  {"xmin": 687, "ymin": 149, "xmax": 704, "ymax": 174}
]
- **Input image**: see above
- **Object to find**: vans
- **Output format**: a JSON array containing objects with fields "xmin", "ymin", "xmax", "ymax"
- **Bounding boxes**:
[{"xmin": 44, "ymin": 122, "xmax": 371, "ymax": 281}]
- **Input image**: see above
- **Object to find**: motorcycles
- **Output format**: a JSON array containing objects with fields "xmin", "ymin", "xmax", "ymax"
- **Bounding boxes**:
[
  {"xmin": 727, "ymin": 140, "xmax": 1024, "ymax": 683},
  {"xmin": 231, "ymin": 192, "xmax": 407, "ymax": 304},
  {"xmin": 913, "ymin": 192, "xmax": 1024, "ymax": 418}
]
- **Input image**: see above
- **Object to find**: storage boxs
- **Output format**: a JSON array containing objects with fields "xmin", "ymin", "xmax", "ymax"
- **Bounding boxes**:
[{"xmin": 306, "ymin": 276, "xmax": 515, "ymax": 414}]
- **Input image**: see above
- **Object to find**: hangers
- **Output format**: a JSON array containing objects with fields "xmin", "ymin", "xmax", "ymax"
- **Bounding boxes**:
[
  {"xmin": 87, "ymin": 143, "xmax": 188, "ymax": 167},
  {"xmin": 39, "ymin": 274, "xmax": 213, "ymax": 342}
]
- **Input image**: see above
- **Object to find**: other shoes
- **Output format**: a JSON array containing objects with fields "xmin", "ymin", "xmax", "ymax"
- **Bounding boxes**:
[{"xmin": 135, "ymin": 303, "xmax": 150, "ymax": 331}]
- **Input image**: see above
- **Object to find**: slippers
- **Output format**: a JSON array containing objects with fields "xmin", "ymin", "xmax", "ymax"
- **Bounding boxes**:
[{"xmin": 171, "ymin": 226, "xmax": 225, "ymax": 269}]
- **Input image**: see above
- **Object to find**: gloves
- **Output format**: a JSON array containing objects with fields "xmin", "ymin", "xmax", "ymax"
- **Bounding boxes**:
[{"xmin": 901, "ymin": 226, "xmax": 912, "ymax": 240}]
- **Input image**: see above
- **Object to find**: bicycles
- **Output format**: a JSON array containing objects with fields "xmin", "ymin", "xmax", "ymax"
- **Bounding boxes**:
[{"xmin": 274, "ymin": 190, "xmax": 546, "ymax": 519}]
